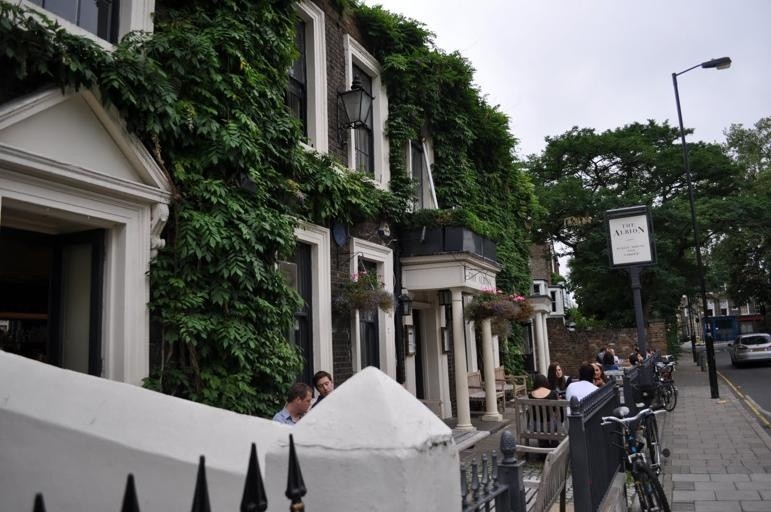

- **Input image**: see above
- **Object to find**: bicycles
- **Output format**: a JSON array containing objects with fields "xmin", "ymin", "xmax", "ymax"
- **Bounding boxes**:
[
  {"xmin": 602, "ymin": 399, "xmax": 673, "ymax": 511},
  {"xmin": 656, "ymin": 354, "xmax": 679, "ymax": 411}
]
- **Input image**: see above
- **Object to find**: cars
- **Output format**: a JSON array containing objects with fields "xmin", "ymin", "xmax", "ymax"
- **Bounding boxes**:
[{"xmin": 729, "ymin": 332, "xmax": 771, "ymax": 368}]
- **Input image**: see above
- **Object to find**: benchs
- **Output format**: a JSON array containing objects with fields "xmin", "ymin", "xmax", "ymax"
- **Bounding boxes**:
[{"xmin": 512, "ymin": 396, "xmax": 571, "ymax": 458}]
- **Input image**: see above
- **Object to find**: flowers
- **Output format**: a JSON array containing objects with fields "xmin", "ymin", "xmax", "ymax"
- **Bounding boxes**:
[
  {"xmin": 463, "ymin": 287, "xmax": 535, "ymax": 324},
  {"xmin": 333, "ymin": 271, "xmax": 399, "ymax": 318}
]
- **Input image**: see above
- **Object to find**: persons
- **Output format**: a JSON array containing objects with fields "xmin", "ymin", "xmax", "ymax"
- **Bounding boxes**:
[
  {"xmin": 271, "ymin": 381, "xmax": 314, "ymax": 426},
  {"xmin": 311, "ymin": 370, "xmax": 334, "ymax": 410},
  {"xmin": 530, "ymin": 340, "xmax": 657, "ymax": 460}
]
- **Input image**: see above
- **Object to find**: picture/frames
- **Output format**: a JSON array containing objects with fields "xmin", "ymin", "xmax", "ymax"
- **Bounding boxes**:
[
  {"xmin": 441, "ymin": 327, "xmax": 451, "ymax": 352},
  {"xmin": 405, "ymin": 325, "xmax": 416, "ymax": 355}
]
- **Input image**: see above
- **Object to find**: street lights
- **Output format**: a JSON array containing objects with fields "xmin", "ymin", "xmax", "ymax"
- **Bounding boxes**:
[{"xmin": 672, "ymin": 52, "xmax": 734, "ymax": 399}]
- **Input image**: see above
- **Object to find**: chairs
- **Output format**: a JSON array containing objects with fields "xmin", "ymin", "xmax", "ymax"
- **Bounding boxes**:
[
  {"xmin": 468, "ymin": 370, "xmax": 506, "ymax": 415},
  {"xmin": 495, "ymin": 365, "xmax": 528, "ymax": 408}
]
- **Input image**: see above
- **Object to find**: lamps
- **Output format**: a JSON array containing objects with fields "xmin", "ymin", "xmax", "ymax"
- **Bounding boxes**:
[
  {"xmin": 398, "ymin": 294, "xmax": 412, "ymax": 315},
  {"xmin": 437, "ymin": 290, "xmax": 451, "ymax": 306},
  {"xmin": 336, "ymin": 75, "xmax": 375, "ymax": 150}
]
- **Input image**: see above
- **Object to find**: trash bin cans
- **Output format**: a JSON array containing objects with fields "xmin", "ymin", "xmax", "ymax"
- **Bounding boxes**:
[{"xmin": 701, "ymin": 351, "xmax": 707, "ymax": 371}]
